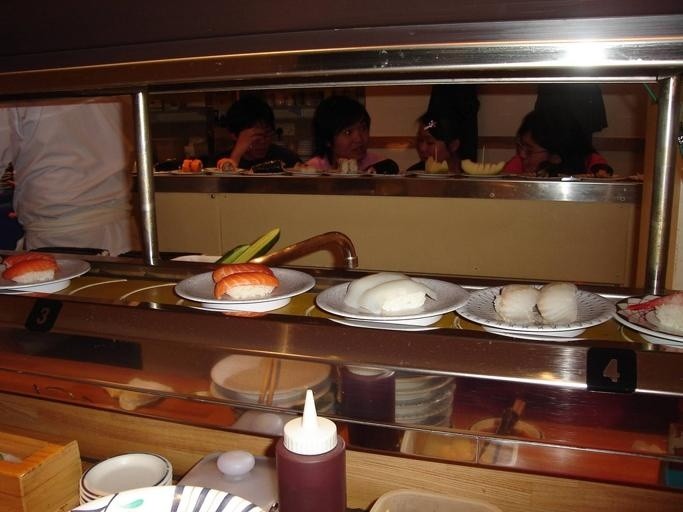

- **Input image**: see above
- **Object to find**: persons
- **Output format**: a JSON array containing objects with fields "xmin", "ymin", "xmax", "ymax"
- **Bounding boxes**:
[
  {"xmin": 497, "ymin": 103, "xmax": 613, "ymax": 177},
  {"xmin": 406, "ymin": 108, "xmax": 463, "ymax": 173},
  {"xmin": 305, "ymin": 94, "xmax": 400, "ymax": 174},
  {"xmin": 210, "ymin": 96, "xmax": 303, "ymax": 173},
  {"xmin": 0, "ymin": 95, "xmax": 143, "ymax": 258}
]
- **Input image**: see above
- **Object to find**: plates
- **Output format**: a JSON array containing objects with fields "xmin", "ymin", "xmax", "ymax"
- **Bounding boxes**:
[
  {"xmin": 208, "ymin": 352, "xmax": 335, "ymax": 418},
  {"xmin": 315, "ymin": 276, "xmax": 470, "ymax": 327},
  {"xmin": 152, "ymin": 168, "xmax": 643, "ymax": 183},
  {"xmin": 612, "ymin": 289, "xmax": 682, "ymax": 348},
  {"xmin": 0, "ymin": 258, "xmax": 90, "ymax": 294},
  {"xmin": 389, "ymin": 372, "xmax": 456, "ymax": 430},
  {"xmin": 71, "ymin": 483, "xmax": 266, "ymax": 512},
  {"xmin": 274, "ymin": 142, "xmax": 312, "ymax": 163},
  {"xmin": 456, "ymin": 282, "xmax": 616, "ymax": 338},
  {"xmin": 80, "ymin": 452, "xmax": 172, "ymax": 505},
  {"xmin": 467, "ymin": 417, "xmax": 545, "ymax": 446},
  {"xmin": 174, "ymin": 266, "xmax": 315, "ymax": 312}
]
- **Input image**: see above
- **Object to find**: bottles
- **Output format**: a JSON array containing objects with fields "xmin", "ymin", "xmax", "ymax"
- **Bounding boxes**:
[
  {"xmin": 338, "ymin": 362, "xmax": 393, "ymax": 425},
  {"xmin": 275, "ymin": 388, "xmax": 346, "ymax": 511}
]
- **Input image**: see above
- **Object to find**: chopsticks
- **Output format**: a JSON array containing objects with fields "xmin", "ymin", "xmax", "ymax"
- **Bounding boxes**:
[{"xmin": 258, "ymin": 357, "xmax": 281, "ymax": 408}]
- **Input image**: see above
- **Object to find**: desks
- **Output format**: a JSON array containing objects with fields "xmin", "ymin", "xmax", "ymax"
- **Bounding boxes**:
[{"xmin": 1, "ymin": 393, "xmax": 683, "ymax": 512}]
